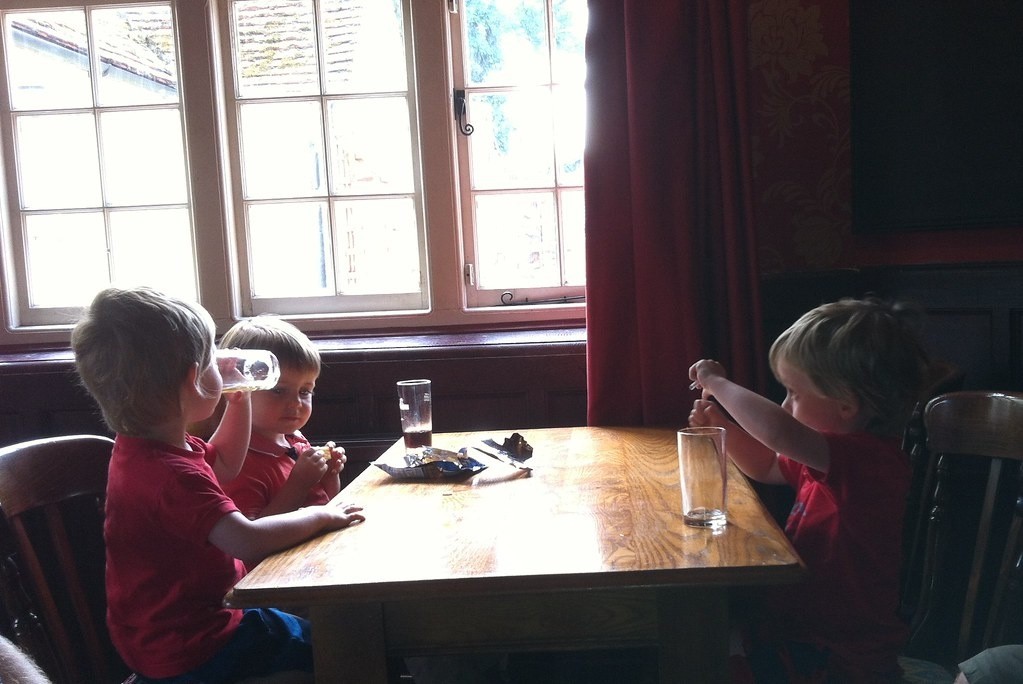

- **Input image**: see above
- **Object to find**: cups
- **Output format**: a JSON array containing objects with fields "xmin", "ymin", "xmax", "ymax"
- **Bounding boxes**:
[
  {"xmin": 677, "ymin": 426, "xmax": 728, "ymax": 528},
  {"xmin": 216, "ymin": 349, "xmax": 280, "ymax": 394},
  {"xmin": 397, "ymin": 378, "xmax": 433, "ymax": 448}
]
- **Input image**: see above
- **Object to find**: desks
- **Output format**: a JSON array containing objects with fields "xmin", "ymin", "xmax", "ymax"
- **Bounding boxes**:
[{"xmin": 224, "ymin": 422, "xmax": 804, "ymax": 681}]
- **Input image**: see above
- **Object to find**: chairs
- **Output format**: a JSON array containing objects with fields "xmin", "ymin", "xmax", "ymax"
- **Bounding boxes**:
[
  {"xmin": 0, "ymin": 435, "xmax": 134, "ymax": 684},
  {"xmin": 859, "ymin": 388, "xmax": 1022, "ymax": 684}
]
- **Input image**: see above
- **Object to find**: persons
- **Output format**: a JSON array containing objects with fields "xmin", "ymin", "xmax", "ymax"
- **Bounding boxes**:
[
  {"xmin": 202, "ymin": 315, "xmax": 349, "ymax": 577},
  {"xmin": 69, "ymin": 282, "xmax": 366, "ymax": 683},
  {"xmin": 687, "ymin": 299, "xmax": 914, "ymax": 684},
  {"xmin": 953, "ymin": 644, "xmax": 1023, "ymax": 684}
]
